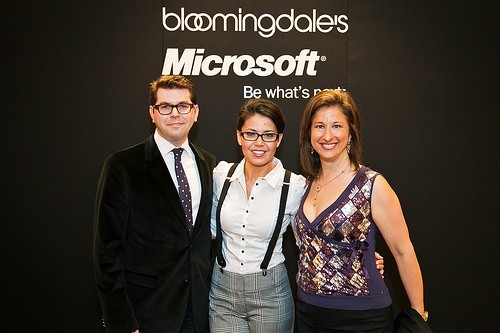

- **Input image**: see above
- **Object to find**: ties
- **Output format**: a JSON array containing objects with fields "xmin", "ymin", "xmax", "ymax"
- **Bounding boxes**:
[{"xmin": 172, "ymin": 148, "xmax": 193, "ymax": 241}]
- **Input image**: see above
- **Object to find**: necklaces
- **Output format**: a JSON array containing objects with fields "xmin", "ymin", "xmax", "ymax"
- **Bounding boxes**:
[{"xmin": 313, "ymin": 162, "xmax": 352, "ymax": 208}]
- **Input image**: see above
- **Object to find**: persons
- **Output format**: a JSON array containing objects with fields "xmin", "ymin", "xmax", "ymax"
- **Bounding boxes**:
[
  {"xmin": 93, "ymin": 76, "xmax": 218, "ymax": 333},
  {"xmin": 291, "ymin": 90, "xmax": 430, "ymax": 333},
  {"xmin": 209, "ymin": 100, "xmax": 385, "ymax": 333}
]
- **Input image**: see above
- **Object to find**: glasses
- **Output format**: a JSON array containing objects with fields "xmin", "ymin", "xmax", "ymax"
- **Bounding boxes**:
[
  {"xmin": 154, "ymin": 102, "xmax": 194, "ymax": 115},
  {"xmin": 240, "ymin": 131, "xmax": 279, "ymax": 142}
]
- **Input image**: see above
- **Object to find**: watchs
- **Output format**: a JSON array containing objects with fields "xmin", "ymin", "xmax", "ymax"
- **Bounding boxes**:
[{"xmin": 419, "ymin": 310, "xmax": 428, "ymax": 322}]
